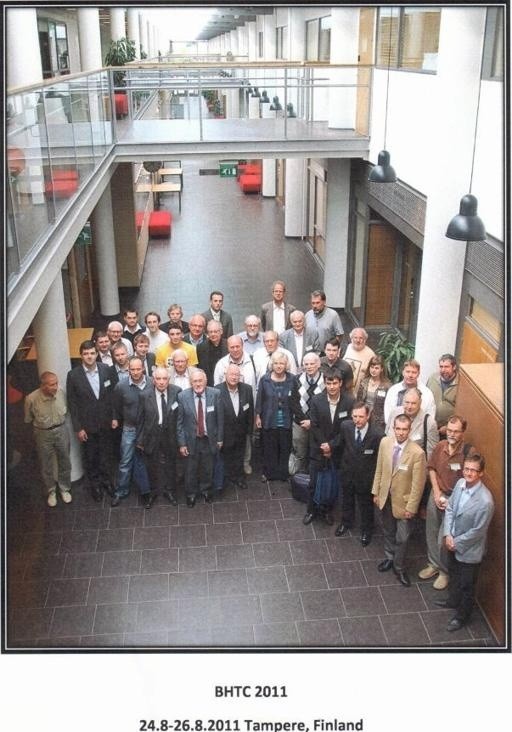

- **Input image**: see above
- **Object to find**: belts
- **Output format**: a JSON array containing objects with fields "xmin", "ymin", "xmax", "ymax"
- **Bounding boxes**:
[
  {"xmin": 193, "ymin": 435, "xmax": 207, "ymax": 440},
  {"xmin": 35, "ymin": 422, "xmax": 65, "ymax": 431},
  {"xmin": 440, "ymin": 488, "xmax": 453, "ymax": 498}
]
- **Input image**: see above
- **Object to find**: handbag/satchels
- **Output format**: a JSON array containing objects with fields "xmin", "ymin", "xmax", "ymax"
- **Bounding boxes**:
[
  {"xmin": 291, "ymin": 468, "xmax": 313, "ymax": 504},
  {"xmin": 314, "ymin": 454, "xmax": 339, "ymax": 506}
]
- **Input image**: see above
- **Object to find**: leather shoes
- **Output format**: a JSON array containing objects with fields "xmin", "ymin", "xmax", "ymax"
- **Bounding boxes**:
[
  {"xmin": 90, "ymin": 476, "xmax": 249, "ymax": 509},
  {"xmin": 319, "ymin": 509, "xmax": 334, "ymax": 525},
  {"xmin": 360, "ymin": 527, "xmax": 371, "ymax": 547},
  {"xmin": 377, "ymin": 557, "xmax": 394, "ymax": 573},
  {"xmin": 393, "ymin": 565, "xmax": 411, "ymax": 588},
  {"xmin": 446, "ymin": 612, "xmax": 471, "ymax": 632},
  {"xmin": 303, "ymin": 507, "xmax": 317, "ymax": 525},
  {"xmin": 336, "ymin": 520, "xmax": 349, "ymax": 536},
  {"xmin": 431, "ymin": 596, "xmax": 460, "ymax": 609}
]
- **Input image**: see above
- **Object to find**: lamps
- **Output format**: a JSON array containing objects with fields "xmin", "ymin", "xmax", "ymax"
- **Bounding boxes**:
[
  {"xmin": 445, "ymin": 7, "xmax": 488, "ymax": 241},
  {"xmin": 369, "ymin": 7, "xmax": 398, "ymax": 183},
  {"xmin": 218, "ymin": 68, "xmax": 296, "ymax": 118}
]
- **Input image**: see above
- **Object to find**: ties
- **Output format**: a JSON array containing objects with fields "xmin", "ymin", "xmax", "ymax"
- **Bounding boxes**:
[
  {"xmin": 310, "ymin": 377, "xmax": 315, "ymax": 385},
  {"xmin": 159, "ymin": 392, "xmax": 170, "ymax": 433},
  {"xmin": 194, "ymin": 393, "xmax": 205, "ymax": 438},
  {"xmin": 355, "ymin": 428, "xmax": 362, "ymax": 449},
  {"xmin": 388, "ymin": 443, "xmax": 401, "ymax": 497}
]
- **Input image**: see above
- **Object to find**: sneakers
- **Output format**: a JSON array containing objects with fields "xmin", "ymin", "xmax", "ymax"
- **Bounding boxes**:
[
  {"xmin": 432, "ymin": 570, "xmax": 450, "ymax": 591},
  {"xmin": 260, "ymin": 473, "xmax": 269, "ymax": 483},
  {"xmin": 47, "ymin": 491, "xmax": 58, "ymax": 506},
  {"xmin": 418, "ymin": 565, "xmax": 439, "ymax": 579},
  {"xmin": 60, "ymin": 489, "xmax": 72, "ymax": 504},
  {"xmin": 242, "ymin": 462, "xmax": 253, "ymax": 475}
]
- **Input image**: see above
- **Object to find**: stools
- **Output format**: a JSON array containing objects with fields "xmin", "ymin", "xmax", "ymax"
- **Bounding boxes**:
[
  {"xmin": 114, "ymin": 93, "xmax": 128, "ymax": 120},
  {"xmin": 135, "ymin": 211, "xmax": 145, "ymax": 233},
  {"xmin": 149, "ymin": 210, "xmax": 172, "ymax": 239},
  {"xmin": 43, "ymin": 168, "xmax": 81, "ymax": 201},
  {"xmin": 237, "ymin": 164, "xmax": 261, "ymax": 195}
]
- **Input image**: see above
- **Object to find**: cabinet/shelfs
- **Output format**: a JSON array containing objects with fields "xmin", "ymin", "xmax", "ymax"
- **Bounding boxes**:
[{"xmin": 454, "ymin": 362, "xmax": 505, "ymax": 646}]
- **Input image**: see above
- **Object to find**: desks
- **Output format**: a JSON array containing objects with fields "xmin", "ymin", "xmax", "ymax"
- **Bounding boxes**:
[
  {"xmin": 136, "ymin": 184, "xmax": 152, "ymax": 192},
  {"xmin": 152, "ymin": 182, "xmax": 182, "ymax": 215},
  {"xmin": 24, "ymin": 327, "xmax": 95, "ymax": 361},
  {"xmin": 152, "ymin": 168, "xmax": 184, "ymax": 193}
]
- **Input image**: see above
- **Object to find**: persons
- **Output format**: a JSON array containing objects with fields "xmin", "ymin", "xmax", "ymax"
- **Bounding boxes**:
[{"xmin": 22, "ymin": 280, "xmax": 497, "ymax": 633}]
